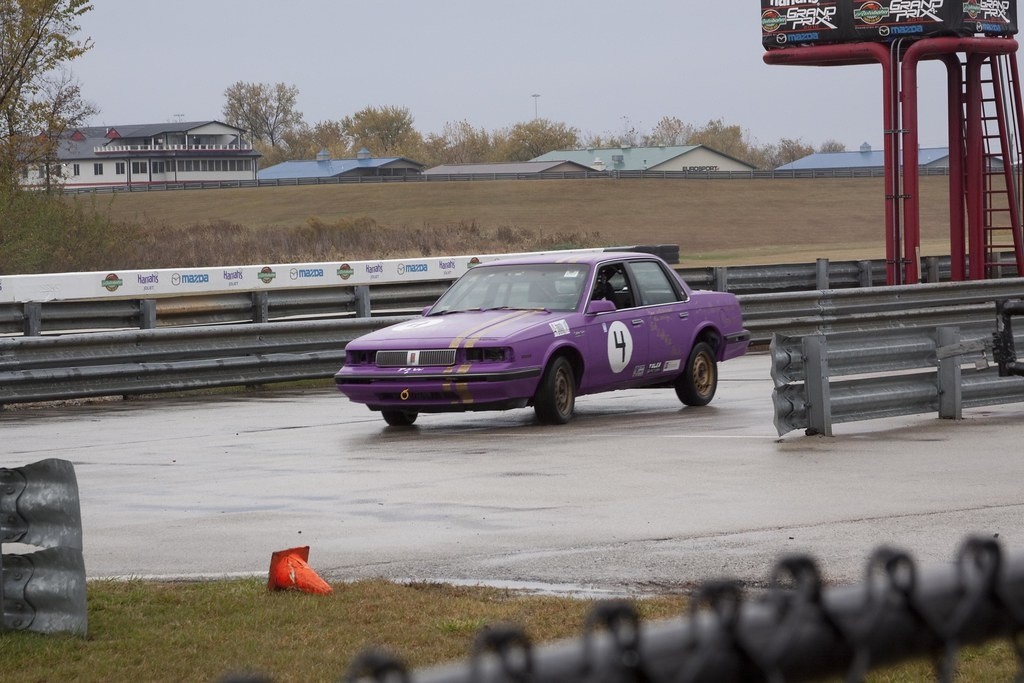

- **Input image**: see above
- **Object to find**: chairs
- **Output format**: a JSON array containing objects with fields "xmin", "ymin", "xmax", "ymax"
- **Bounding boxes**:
[
  {"xmin": 599, "ymin": 281, "xmax": 618, "ymax": 311},
  {"xmin": 528, "ymin": 279, "xmax": 558, "ymax": 304}
]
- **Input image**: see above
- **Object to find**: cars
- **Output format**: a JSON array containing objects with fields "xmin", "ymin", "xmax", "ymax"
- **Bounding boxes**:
[{"xmin": 334, "ymin": 248, "xmax": 751, "ymax": 428}]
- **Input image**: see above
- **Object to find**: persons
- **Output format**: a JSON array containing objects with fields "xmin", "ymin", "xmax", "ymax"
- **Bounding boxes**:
[{"xmin": 575, "ymin": 266, "xmax": 617, "ymax": 309}]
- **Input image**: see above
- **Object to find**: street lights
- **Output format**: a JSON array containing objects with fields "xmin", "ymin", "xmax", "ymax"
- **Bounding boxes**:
[{"xmin": 532, "ymin": 93, "xmax": 541, "ymax": 120}]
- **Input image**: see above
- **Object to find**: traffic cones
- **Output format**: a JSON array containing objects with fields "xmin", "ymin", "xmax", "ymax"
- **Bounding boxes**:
[{"xmin": 267, "ymin": 546, "xmax": 332, "ymax": 599}]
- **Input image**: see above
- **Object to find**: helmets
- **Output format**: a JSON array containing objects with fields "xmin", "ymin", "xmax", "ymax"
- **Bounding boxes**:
[{"xmin": 574, "ymin": 264, "xmax": 606, "ymax": 299}]
